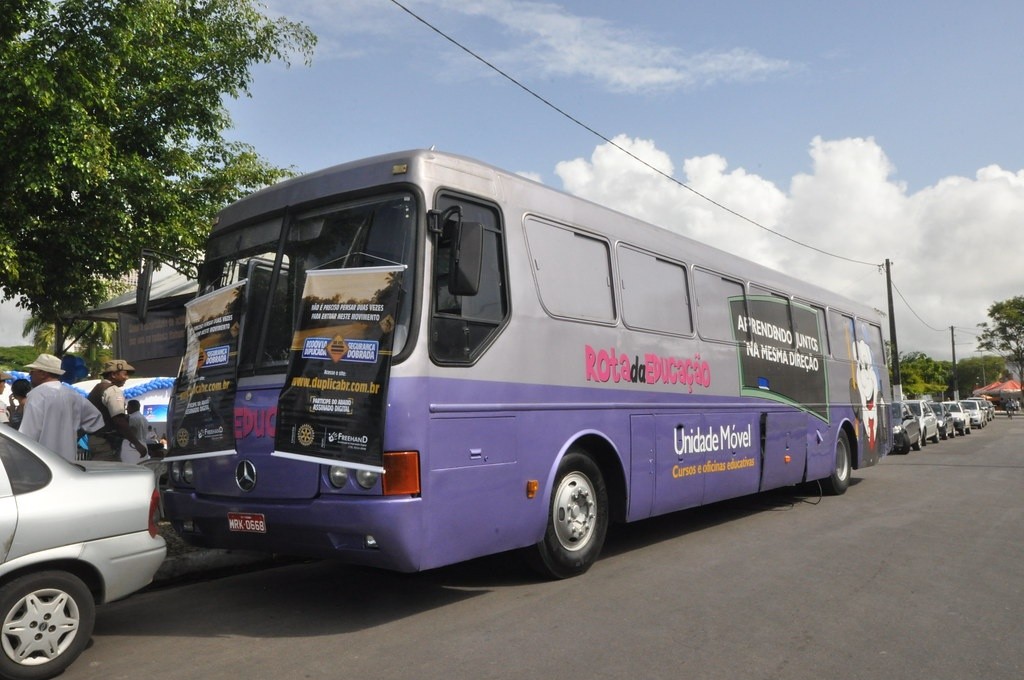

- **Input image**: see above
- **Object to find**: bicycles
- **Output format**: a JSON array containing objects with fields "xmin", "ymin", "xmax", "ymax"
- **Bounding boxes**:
[{"xmin": 1008, "ymin": 407, "xmax": 1012, "ymax": 420}]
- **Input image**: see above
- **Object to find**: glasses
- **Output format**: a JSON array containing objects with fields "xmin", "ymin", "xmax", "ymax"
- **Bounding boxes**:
[{"xmin": 28, "ymin": 369, "xmax": 37, "ymax": 375}]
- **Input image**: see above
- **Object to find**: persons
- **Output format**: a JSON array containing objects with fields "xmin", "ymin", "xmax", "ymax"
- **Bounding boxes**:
[
  {"xmin": 1000, "ymin": 397, "xmax": 1021, "ymax": 414},
  {"xmin": 0, "ymin": 353, "xmax": 167, "ymax": 462}
]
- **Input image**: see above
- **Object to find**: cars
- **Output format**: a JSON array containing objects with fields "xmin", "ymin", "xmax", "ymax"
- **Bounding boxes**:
[
  {"xmin": 892, "ymin": 402, "xmax": 921, "ymax": 454},
  {"xmin": 0, "ymin": 421, "xmax": 167, "ymax": 680},
  {"xmin": 942, "ymin": 402, "xmax": 970, "ymax": 436},
  {"xmin": 957, "ymin": 398, "xmax": 996, "ymax": 429},
  {"xmin": 929, "ymin": 402, "xmax": 955, "ymax": 440},
  {"xmin": 903, "ymin": 400, "xmax": 939, "ymax": 446}
]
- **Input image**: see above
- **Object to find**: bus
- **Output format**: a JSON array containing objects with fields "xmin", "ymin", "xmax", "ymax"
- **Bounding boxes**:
[{"xmin": 158, "ymin": 149, "xmax": 891, "ymax": 582}]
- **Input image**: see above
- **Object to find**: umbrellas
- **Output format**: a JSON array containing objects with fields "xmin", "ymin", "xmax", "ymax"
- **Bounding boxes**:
[{"xmin": 973, "ymin": 380, "xmax": 1021, "ymax": 393}]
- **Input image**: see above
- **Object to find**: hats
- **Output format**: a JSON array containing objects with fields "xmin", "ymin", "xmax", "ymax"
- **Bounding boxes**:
[
  {"xmin": 98, "ymin": 360, "xmax": 135, "ymax": 375},
  {"xmin": 0, "ymin": 369, "xmax": 12, "ymax": 380},
  {"xmin": 23, "ymin": 353, "xmax": 66, "ymax": 375}
]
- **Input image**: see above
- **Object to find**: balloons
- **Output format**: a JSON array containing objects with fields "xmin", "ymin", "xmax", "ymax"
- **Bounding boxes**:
[{"xmin": 5, "ymin": 371, "xmax": 175, "ymax": 400}]
